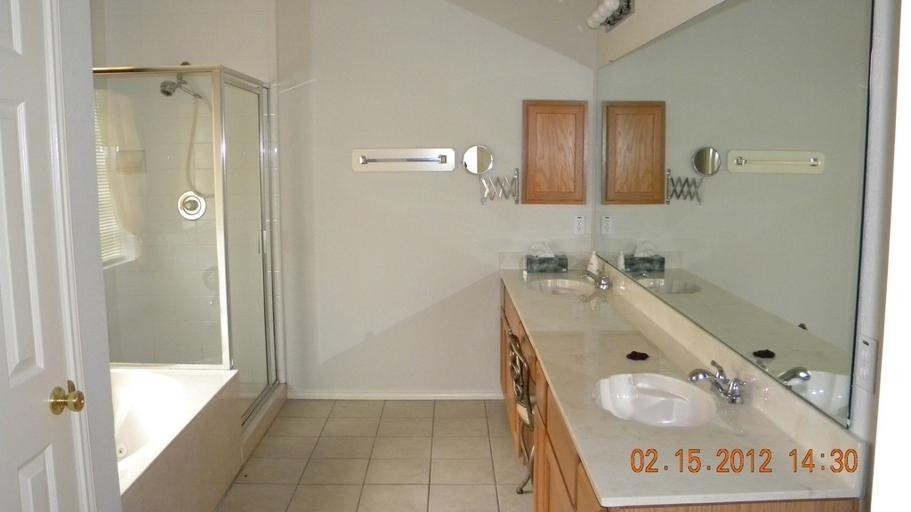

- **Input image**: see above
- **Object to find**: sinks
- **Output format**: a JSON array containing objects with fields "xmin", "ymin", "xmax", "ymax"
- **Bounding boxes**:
[
  {"xmin": 527, "ymin": 279, "xmax": 593, "ymax": 296},
  {"xmin": 591, "ymin": 372, "xmax": 719, "ymax": 429},
  {"xmin": 636, "ymin": 278, "xmax": 702, "ymax": 295},
  {"xmin": 773, "ymin": 370, "xmax": 851, "ymax": 426}
]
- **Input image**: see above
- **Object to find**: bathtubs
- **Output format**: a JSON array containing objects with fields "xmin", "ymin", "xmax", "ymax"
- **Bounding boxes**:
[{"xmin": 109, "ymin": 361, "xmax": 242, "ymax": 512}]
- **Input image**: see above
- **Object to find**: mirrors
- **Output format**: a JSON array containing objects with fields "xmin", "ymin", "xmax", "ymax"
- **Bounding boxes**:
[
  {"xmin": 692, "ymin": 147, "xmax": 721, "ymax": 177},
  {"xmin": 594, "ymin": 0, "xmax": 874, "ymax": 430},
  {"xmin": 462, "ymin": 145, "xmax": 493, "ymax": 175}
]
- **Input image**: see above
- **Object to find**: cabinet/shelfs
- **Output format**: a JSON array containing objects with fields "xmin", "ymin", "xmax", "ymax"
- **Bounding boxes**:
[
  {"xmin": 535, "ymin": 366, "xmax": 549, "ymax": 511},
  {"xmin": 578, "ymin": 463, "xmax": 607, "ymax": 510},
  {"xmin": 522, "ymin": 100, "xmax": 588, "ymax": 204},
  {"xmin": 549, "ymin": 385, "xmax": 577, "ymax": 512},
  {"xmin": 602, "ymin": 101, "xmax": 665, "ymax": 205},
  {"xmin": 501, "ymin": 280, "xmax": 535, "ymax": 466}
]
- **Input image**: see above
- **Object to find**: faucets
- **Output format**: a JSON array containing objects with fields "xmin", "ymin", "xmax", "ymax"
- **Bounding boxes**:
[
  {"xmin": 576, "ymin": 290, "xmax": 608, "ymax": 304},
  {"xmin": 582, "ymin": 270, "xmax": 609, "ymax": 289},
  {"xmin": 628, "ymin": 272, "xmax": 648, "ymax": 280},
  {"xmin": 775, "ymin": 366, "xmax": 810, "ymax": 383},
  {"xmin": 688, "ymin": 360, "xmax": 745, "ymax": 403}
]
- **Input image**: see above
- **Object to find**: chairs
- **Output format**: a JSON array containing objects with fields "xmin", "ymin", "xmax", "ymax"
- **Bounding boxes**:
[{"xmin": 508, "ymin": 334, "xmax": 535, "ymax": 494}]
